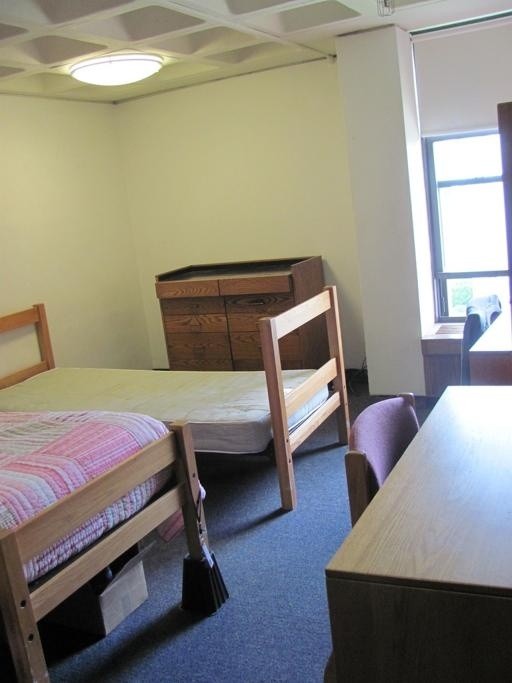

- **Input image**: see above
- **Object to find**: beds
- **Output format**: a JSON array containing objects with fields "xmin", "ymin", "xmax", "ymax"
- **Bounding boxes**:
[
  {"xmin": 0, "ymin": 285, "xmax": 351, "ymax": 511},
  {"xmin": 0, "ymin": 412, "xmax": 229, "ymax": 683}
]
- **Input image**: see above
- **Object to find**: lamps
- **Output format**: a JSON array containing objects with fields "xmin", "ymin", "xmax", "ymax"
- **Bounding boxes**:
[{"xmin": 67, "ymin": 54, "xmax": 164, "ymax": 86}]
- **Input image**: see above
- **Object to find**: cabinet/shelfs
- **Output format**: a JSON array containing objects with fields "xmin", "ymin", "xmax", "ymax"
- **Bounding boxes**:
[
  {"xmin": 156, "ymin": 257, "xmax": 333, "ymax": 390},
  {"xmin": 325, "ymin": 386, "xmax": 512, "ymax": 683},
  {"xmin": 468, "ymin": 102, "xmax": 512, "ymax": 385}
]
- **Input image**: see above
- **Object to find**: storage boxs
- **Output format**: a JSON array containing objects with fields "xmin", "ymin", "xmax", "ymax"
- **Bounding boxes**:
[{"xmin": 51, "ymin": 539, "xmax": 157, "ymax": 635}]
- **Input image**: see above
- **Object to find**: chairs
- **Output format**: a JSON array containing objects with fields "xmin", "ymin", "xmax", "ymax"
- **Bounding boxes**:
[
  {"xmin": 345, "ymin": 392, "xmax": 420, "ymax": 527},
  {"xmin": 460, "ymin": 295, "xmax": 501, "ymax": 385}
]
